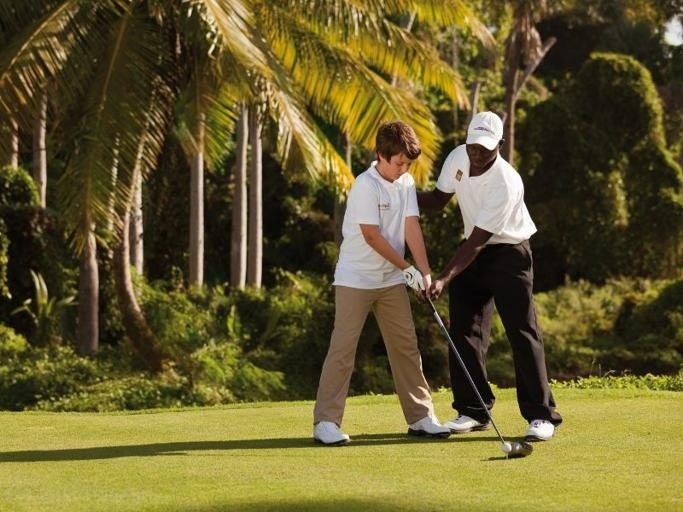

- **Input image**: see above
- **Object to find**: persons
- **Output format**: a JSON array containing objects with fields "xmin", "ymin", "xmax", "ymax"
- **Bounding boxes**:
[
  {"xmin": 313, "ymin": 121, "xmax": 451, "ymax": 446},
  {"xmin": 416, "ymin": 111, "xmax": 562, "ymax": 442}
]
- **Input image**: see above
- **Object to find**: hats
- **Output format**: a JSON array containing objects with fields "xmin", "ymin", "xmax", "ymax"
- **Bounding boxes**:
[{"xmin": 466, "ymin": 110, "xmax": 504, "ymax": 151}]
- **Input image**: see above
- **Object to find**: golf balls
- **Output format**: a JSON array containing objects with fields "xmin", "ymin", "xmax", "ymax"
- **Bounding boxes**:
[{"xmin": 502, "ymin": 443, "xmax": 512, "ymax": 453}]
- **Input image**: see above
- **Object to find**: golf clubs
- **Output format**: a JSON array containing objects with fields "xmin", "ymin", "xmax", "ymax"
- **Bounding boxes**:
[{"xmin": 423, "ymin": 289, "xmax": 532, "ymax": 456}]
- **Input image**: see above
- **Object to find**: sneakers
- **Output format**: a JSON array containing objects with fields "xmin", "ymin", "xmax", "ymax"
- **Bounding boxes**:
[
  {"xmin": 525, "ymin": 418, "xmax": 555, "ymax": 442},
  {"xmin": 444, "ymin": 414, "xmax": 492, "ymax": 433},
  {"xmin": 409, "ymin": 414, "xmax": 450, "ymax": 438},
  {"xmin": 313, "ymin": 420, "xmax": 349, "ymax": 445}
]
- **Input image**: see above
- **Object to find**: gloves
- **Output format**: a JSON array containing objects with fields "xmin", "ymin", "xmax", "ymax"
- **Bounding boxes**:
[{"xmin": 403, "ymin": 265, "xmax": 425, "ymax": 292}]
